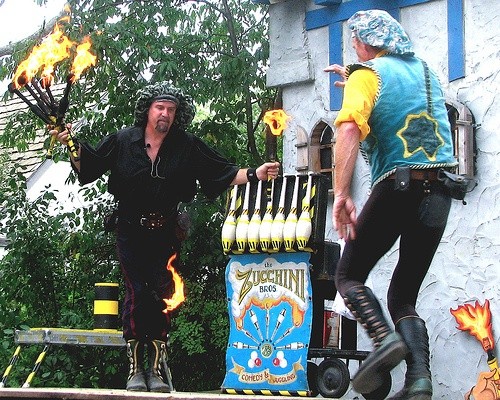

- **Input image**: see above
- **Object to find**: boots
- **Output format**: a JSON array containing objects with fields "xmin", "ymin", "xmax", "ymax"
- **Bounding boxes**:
[
  {"xmin": 146, "ymin": 339, "xmax": 170, "ymax": 393},
  {"xmin": 344, "ymin": 285, "xmax": 410, "ymax": 393},
  {"xmin": 127, "ymin": 337, "xmax": 148, "ymax": 392},
  {"xmin": 385, "ymin": 315, "xmax": 433, "ymax": 400}
]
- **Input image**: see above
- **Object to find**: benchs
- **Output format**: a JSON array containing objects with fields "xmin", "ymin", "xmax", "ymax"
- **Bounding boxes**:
[{"xmin": 0, "ymin": 328, "xmax": 177, "ymax": 394}]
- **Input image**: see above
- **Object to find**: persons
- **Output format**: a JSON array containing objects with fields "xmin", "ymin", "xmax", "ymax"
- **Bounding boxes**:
[
  {"xmin": 47, "ymin": 79, "xmax": 280, "ymax": 392},
  {"xmin": 463, "ymin": 368, "xmax": 499, "ymax": 400},
  {"xmin": 322, "ymin": 9, "xmax": 458, "ymax": 400}
]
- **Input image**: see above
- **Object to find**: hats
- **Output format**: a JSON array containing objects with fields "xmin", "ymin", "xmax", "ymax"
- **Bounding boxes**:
[
  {"xmin": 134, "ymin": 79, "xmax": 195, "ymax": 130},
  {"xmin": 347, "ymin": 9, "xmax": 416, "ymax": 55}
]
